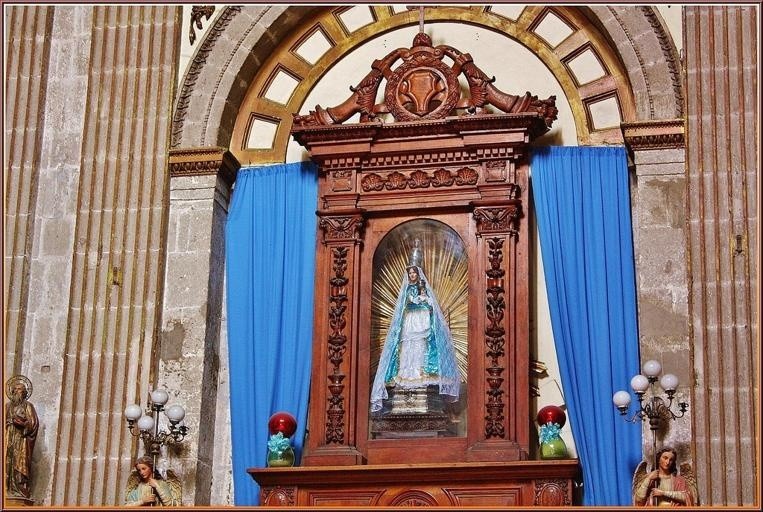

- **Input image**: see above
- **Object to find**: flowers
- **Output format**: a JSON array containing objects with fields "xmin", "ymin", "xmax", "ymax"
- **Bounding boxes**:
[
  {"xmin": 267, "ymin": 432, "xmax": 292, "ymax": 458},
  {"xmin": 538, "ymin": 421, "xmax": 561, "ymax": 444}
]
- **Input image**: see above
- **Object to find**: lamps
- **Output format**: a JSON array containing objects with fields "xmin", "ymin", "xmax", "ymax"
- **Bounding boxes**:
[
  {"xmin": 123, "ymin": 387, "xmax": 188, "ymax": 505},
  {"xmin": 612, "ymin": 359, "xmax": 689, "ymax": 508}
]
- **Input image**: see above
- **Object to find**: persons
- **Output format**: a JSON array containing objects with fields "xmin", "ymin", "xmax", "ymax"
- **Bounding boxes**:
[
  {"xmin": 121, "ymin": 457, "xmax": 179, "ymax": 506},
  {"xmin": 392, "ymin": 266, "xmax": 438, "ymax": 385},
  {"xmin": 631, "ymin": 445, "xmax": 698, "ymax": 507},
  {"xmin": 5, "ymin": 382, "xmax": 36, "ymax": 502}
]
